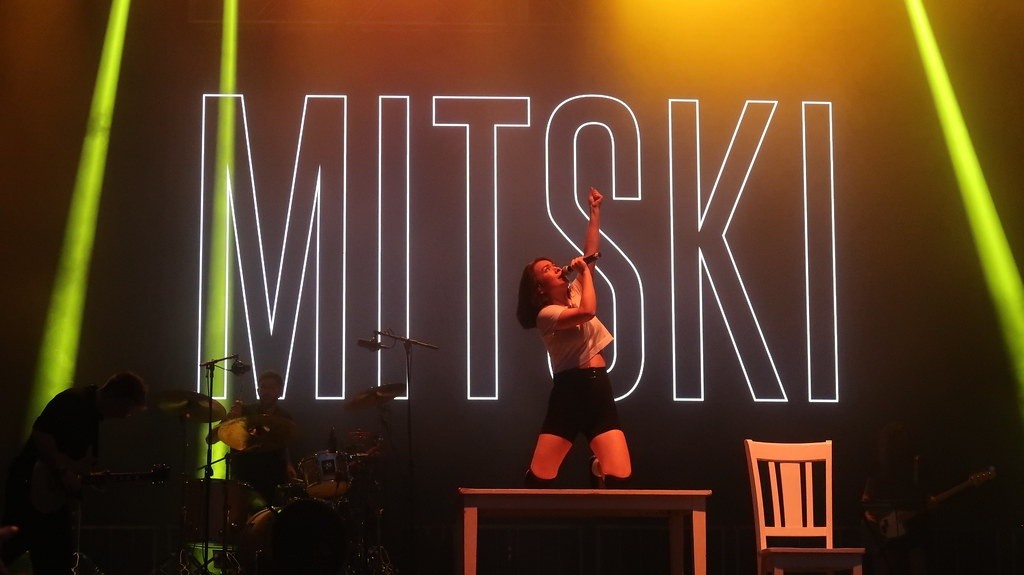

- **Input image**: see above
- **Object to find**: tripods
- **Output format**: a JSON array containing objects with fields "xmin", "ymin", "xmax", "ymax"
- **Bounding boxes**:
[
  {"xmin": 340, "ymin": 504, "xmax": 394, "ymax": 575},
  {"xmin": 150, "ymin": 354, "xmax": 249, "ymax": 575},
  {"xmin": 68, "ymin": 465, "xmax": 171, "ymax": 575}
]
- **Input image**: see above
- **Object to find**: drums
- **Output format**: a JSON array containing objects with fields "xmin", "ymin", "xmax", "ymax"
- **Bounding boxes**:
[
  {"xmin": 180, "ymin": 477, "xmax": 250, "ymax": 543},
  {"xmin": 238, "ymin": 497, "xmax": 347, "ymax": 575},
  {"xmin": 299, "ymin": 450, "xmax": 353, "ymax": 501}
]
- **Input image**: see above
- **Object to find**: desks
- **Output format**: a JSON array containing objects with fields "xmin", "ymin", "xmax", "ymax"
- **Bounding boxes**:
[
  {"xmin": 459, "ymin": 486, "xmax": 714, "ymax": 575},
  {"xmin": 70, "ymin": 522, "xmax": 159, "ymax": 575}
]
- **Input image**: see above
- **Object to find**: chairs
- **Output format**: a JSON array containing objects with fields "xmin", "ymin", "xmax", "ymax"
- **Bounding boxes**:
[{"xmin": 745, "ymin": 438, "xmax": 866, "ymax": 575}]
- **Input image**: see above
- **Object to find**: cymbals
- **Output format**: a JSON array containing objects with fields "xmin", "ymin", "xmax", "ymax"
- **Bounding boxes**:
[
  {"xmin": 217, "ymin": 413, "xmax": 300, "ymax": 455},
  {"xmin": 154, "ymin": 388, "xmax": 227, "ymax": 424},
  {"xmin": 347, "ymin": 381, "xmax": 408, "ymax": 413}
]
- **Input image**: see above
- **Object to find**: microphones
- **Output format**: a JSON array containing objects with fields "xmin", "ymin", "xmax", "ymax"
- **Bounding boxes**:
[
  {"xmin": 356, "ymin": 338, "xmax": 387, "ymax": 352},
  {"xmin": 230, "ymin": 362, "xmax": 252, "ymax": 374},
  {"xmin": 328, "ymin": 426, "xmax": 337, "ymax": 450},
  {"xmin": 560, "ymin": 253, "xmax": 602, "ymax": 276}
]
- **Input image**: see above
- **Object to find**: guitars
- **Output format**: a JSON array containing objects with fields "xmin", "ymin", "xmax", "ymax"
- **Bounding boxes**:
[
  {"xmin": 30, "ymin": 452, "xmax": 173, "ymax": 518},
  {"xmin": 876, "ymin": 467, "xmax": 997, "ymax": 540}
]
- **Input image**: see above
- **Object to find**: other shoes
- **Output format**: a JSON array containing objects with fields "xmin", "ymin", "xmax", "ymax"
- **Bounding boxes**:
[
  {"xmin": 525, "ymin": 469, "xmax": 538, "ymax": 488},
  {"xmin": 590, "ymin": 455, "xmax": 606, "ymax": 490}
]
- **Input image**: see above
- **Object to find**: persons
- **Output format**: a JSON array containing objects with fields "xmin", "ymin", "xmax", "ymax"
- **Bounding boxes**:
[
  {"xmin": 862, "ymin": 422, "xmax": 940, "ymax": 575},
  {"xmin": 5, "ymin": 372, "xmax": 146, "ymax": 575},
  {"xmin": 206, "ymin": 370, "xmax": 296, "ymax": 504},
  {"xmin": 517, "ymin": 187, "xmax": 633, "ymax": 488}
]
